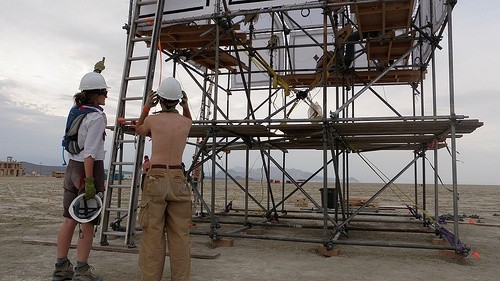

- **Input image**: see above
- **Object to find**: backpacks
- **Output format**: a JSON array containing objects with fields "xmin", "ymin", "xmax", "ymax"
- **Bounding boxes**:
[{"xmin": 63, "ymin": 110, "xmax": 99, "ymax": 155}]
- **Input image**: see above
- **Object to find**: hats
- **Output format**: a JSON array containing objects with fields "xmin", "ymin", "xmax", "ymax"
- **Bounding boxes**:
[
  {"xmin": 156, "ymin": 77, "xmax": 184, "ymax": 100},
  {"xmin": 78, "ymin": 72, "xmax": 111, "ymax": 91}
]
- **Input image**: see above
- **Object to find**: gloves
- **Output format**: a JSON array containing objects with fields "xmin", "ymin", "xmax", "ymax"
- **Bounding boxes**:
[
  {"xmin": 84, "ymin": 177, "xmax": 96, "ymax": 200},
  {"xmin": 94, "ymin": 57, "xmax": 105, "ymax": 71}
]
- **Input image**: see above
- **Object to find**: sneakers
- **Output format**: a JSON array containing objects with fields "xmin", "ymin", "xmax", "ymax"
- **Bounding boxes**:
[
  {"xmin": 52, "ymin": 257, "xmax": 75, "ymax": 281},
  {"xmin": 71, "ymin": 261, "xmax": 102, "ymax": 281}
]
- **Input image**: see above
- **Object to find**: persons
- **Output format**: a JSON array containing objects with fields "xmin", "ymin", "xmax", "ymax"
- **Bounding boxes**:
[
  {"xmin": 137, "ymin": 77, "xmax": 193, "ymax": 281},
  {"xmin": 141, "ymin": 155, "xmax": 150, "ymax": 191},
  {"xmin": 338, "ymin": 23, "xmax": 395, "ymax": 72},
  {"xmin": 52, "ymin": 57, "xmax": 108, "ymax": 281},
  {"xmin": 192, "ymin": 155, "xmax": 201, "ymax": 203},
  {"xmin": 315, "ymin": 50, "xmax": 355, "ymax": 73}
]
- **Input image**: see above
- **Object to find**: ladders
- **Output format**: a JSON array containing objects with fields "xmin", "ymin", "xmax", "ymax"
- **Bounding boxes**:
[
  {"xmin": 98, "ymin": 0, "xmax": 166, "ymax": 253},
  {"xmin": 189, "ymin": 68, "xmax": 214, "ymax": 216}
]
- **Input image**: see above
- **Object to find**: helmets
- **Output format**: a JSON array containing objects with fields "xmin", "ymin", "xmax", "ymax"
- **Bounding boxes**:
[{"xmin": 69, "ymin": 193, "xmax": 103, "ymax": 223}]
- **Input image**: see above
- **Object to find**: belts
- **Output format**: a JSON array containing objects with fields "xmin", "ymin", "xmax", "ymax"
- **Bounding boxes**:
[{"xmin": 146, "ymin": 164, "xmax": 184, "ymax": 169}]
